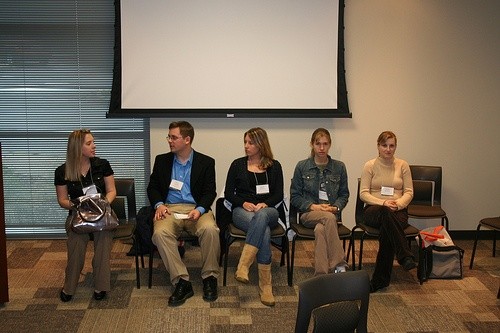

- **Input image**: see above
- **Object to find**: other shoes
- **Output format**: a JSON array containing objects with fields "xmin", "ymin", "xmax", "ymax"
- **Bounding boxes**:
[
  {"xmin": 334, "ymin": 267, "xmax": 346, "ymax": 273},
  {"xmin": 398, "ymin": 257, "xmax": 419, "ymax": 270},
  {"xmin": 369, "ymin": 280, "xmax": 389, "ymax": 293}
]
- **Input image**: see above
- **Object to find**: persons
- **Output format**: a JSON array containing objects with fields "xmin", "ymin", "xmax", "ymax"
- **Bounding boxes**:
[
  {"xmin": 359, "ymin": 131, "xmax": 417, "ymax": 292},
  {"xmin": 223, "ymin": 126, "xmax": 285, "ymax": 306},
  {"xmin": 288, "ymin": 124, "xmax": 349, "ymax": 283},
  {"xmin": 55, "ymin": 129, "xmax": 117, "ymax": 302},
  {"xmin": 147, "ymin": 119, "xmax": 222, "ymax": 305}
]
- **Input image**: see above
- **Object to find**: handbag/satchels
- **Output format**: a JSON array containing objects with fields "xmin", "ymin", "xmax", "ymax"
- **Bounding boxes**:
[
  {"xmin": 71, "ymin": 198, "xmax": 120, "ymax": 233},
  {"xmin": 425, "ymin": 244, "xmax": 464, "ymax": 279},
  {"xmin": 416, "ymin": 225, "xmax": 456, "ymax": 248}
]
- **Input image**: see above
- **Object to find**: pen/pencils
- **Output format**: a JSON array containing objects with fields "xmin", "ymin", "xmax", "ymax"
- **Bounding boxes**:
[{"xmin": 160, "ymin": 209, "xmax": 166, "ymax": 219}]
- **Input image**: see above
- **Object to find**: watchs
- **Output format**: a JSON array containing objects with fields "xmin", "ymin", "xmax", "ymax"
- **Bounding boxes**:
[{"xmin": 336, "ymin": 206, "xmax": 340, "ymax": 212}]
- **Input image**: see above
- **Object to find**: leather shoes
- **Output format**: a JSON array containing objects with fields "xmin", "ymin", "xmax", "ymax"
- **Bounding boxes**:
[
  {"xmin": 94, "ymin": 289, "xmax": 106, "ymax": 300},
  {"xmin": 60, "ymin": 287, "xmax": 72, "ymax": 302},
  {"xmin": 204, "ymin": 275, "xmax": 218, "ymax": 298},
  {"xmin": 168, "ymin": 278, "xmax": 194, "ymax": 304}
]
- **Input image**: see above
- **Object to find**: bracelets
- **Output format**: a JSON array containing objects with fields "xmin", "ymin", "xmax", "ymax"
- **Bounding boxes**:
[{"xmin": 383, "ymin": 200, "xmax": 386, "ymax": 206}]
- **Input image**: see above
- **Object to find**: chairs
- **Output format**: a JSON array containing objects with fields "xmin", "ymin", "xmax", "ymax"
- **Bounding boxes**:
[
  {"xmin": 280, "ymin": 178, "xmax": 353, "ymax": 286},
  {"xmin": 469, "ymin": 217, "xmax": 500, "ymax": 300},
  {"xmin": 90, "ymin": 176, "xmax": 145, "ymax": 270},
  {"xmin": 403, "ymin": 164, "xmax": 449, "ymax": 249},
  {"xmin": 133, "ymin": 175, "xmax": 213, "ymax": 289},
  {"xmin": 347, "ymin": 179, "xmax": 424, "ymax": 286},
  {"xmin": 215, "ymin": 183, "xmax": 293, "ymax": 287},
  {"xmin": 294, "ymin": 269, "xmax": 370, "ymax": 333}
]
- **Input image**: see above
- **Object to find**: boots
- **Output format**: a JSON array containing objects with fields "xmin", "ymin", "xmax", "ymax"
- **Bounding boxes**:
[
  {"xmin": 236, "ymin": 243, "xmax": 258, "ymax": 281},
  {"xmin": 258, "ymin": 262, "xmax": 276, "ymax": 305}
]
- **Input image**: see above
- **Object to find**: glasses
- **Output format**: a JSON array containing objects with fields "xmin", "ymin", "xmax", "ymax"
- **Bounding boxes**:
[{"xmin": 167, "ymin": 135, "xmax": 186, "ymax": 141}]
